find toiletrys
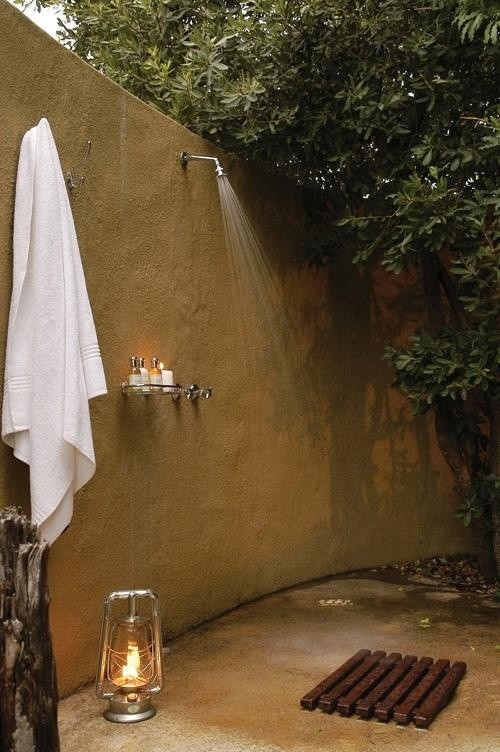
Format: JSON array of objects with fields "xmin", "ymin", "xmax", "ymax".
[
  {"xmin": 128, "ymin": 356, "xmax": 142, "ymax": 392},
  {"xmin": 139, "ymin": 359, "xmax": 149, "ymax": 391},
  {"xmin": 150, "ymin": 358, "xmax": 162, "ymax": 392}
]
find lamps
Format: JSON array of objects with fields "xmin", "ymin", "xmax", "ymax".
[{"xmin": 96, "ymin": 587, "xmax": 164, "ymax": 723}]
